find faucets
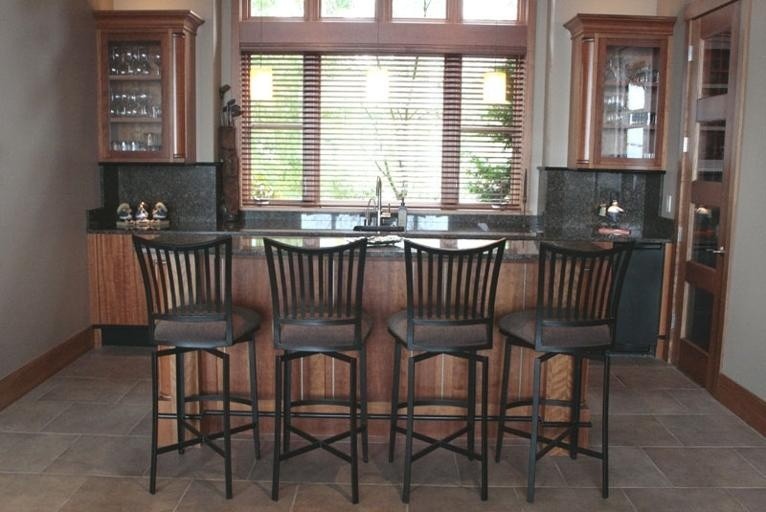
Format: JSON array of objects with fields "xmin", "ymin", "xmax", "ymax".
[{"xmin": 375, "ymin": 175, "xmax": 382, "ymax": 226}]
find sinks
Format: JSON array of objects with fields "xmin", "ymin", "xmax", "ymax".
[{"xmin": 353, "ymin": 225, "xmax": 404, "ymax": 232}]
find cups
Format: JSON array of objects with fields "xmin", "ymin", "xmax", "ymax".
[
  {"xmin": 132, "ymin": 47, "xmax": 140, "ymax": 75},
  {"xmin": 129, "ymin": 89, "xmax": 136, "ymax": 118},
  {"xmin": 120, "ymin": 136, "xmax": 128, "ymax": 151},
  {"xmin": 152, "ymin": 103, "xmax": 161, "ymax": 118},
  {"xmin": 138, "ymin": 47, "xmax": 149, "ymax": 75},
  {"xmin": 112, "ymin": 136, "xmax": 120, "ymax": 151},
  {"xmin": 122, "ymin": 89, "xmax": 129, "ymax": 118},
  {"xmin": 110, "ymin": 88, "xmax": 121, "ymax": 117},
  {"xmin": 111, "ymin": 46, "xmax": 121, "ymax": 75},
  {"xmin": 130, "ymin": 137, "xmax": 138, "ymax": 152},
  {"xmin": 152, "ymin": 48, "xmax": 161, "ymax": 76},
  {"xmin": 136, "ymin": 88, "xmax": 152, "ymax": 118},
  {"xmin": 122, "ymin": 46, "xmax": 132, "ymax": 75}
]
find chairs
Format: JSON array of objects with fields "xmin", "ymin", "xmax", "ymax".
[
  {"xmin": 261, "ymin": 235, "xmax": 375, "ymax": 505},
  {"xmin": 493, "ymin": 238, "xmax": 638, "ymax": 504},
  {"xmin": 386, "ymin": 235, "xmax": 508, "ymax": 503},
  {"xmin": 132, "ymin": 231, "xmax": 264, "ymax": 499}
]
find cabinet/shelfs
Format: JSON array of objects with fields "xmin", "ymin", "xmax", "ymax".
[
  {"xmin": 94, "ymin": 8, "xmax": 205, "ymax": 165},
  {"xmin": 88, "ymin": 229, "xmax": 158, "ymax": 349},
  {"xmin": 565, "ymin": 13, "xmax": 666, "ymax": 169}
]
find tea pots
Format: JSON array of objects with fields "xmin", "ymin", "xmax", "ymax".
[{"xmin": 143, "ymin": 132, "xmax": 159, "ymax": 152}]
